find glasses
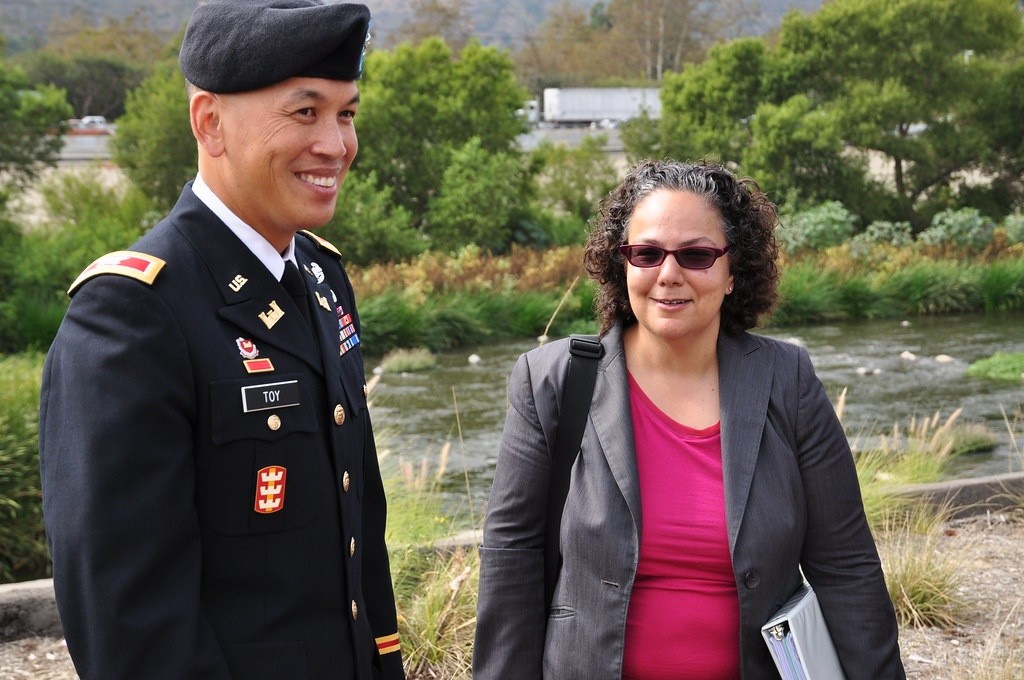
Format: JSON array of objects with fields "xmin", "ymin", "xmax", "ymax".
[{"xmin": 617, "ymin": 243, "xmax": 734, "ymax": 268}]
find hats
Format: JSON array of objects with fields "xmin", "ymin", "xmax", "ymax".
[{"xmin": 179, "ymin": 0, "xmax": 370, "ymax": 93}]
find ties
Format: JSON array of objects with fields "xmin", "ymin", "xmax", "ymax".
[{"xmin": 283, "ymin": 261, "xmax": 320, "ymax": 349}]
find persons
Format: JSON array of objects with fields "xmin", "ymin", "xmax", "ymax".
[
  {"xmin": 468, "ymin": 157, "xmax": 910, "ymax": 680},
  {"xmin": 36, "ymin": 0, "xmax": 408, "ymax": 680}
]
